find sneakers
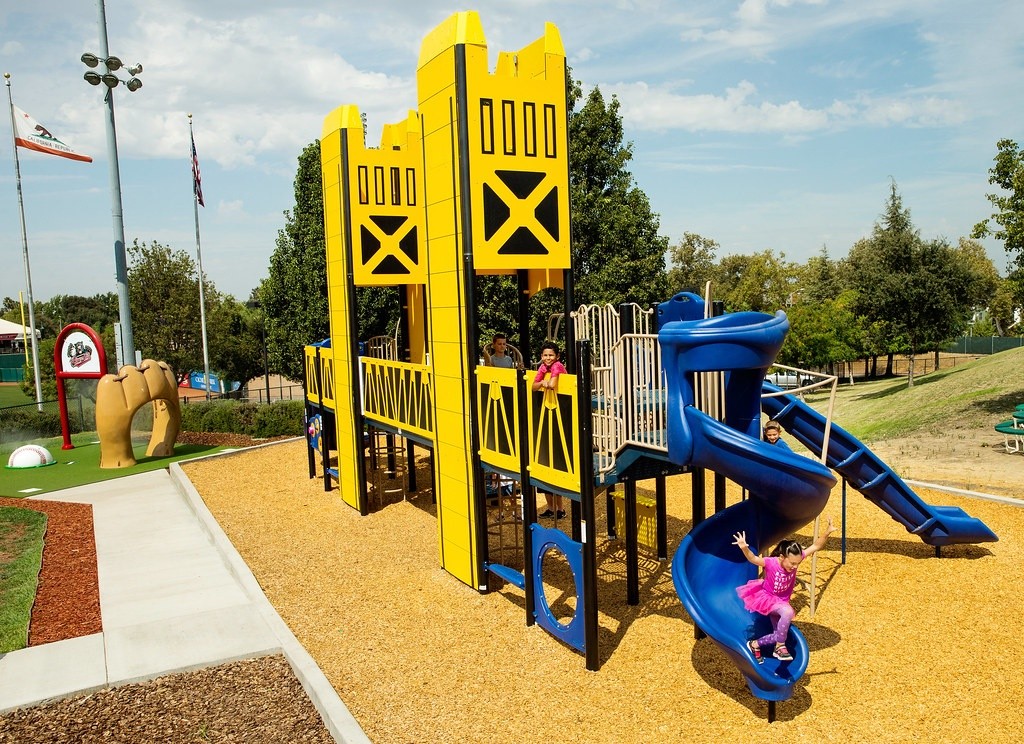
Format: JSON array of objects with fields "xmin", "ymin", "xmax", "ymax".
[
  {"xmin": 538, "ymin": 509, "xmax": 555, "ymax": 519},
  {"xmin": 550, "ymin": 509, "xmax": 566, "ymax": 521},
  {"xmin": 773, "ymin": 644, "xmax": 793, "ymax": 660},
  {"xmin": 746, "ymin": 640, "xmax": 764, "ymax": 664}
]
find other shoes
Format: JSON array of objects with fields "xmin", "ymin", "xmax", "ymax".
[
  {"xmin": 513, "ymin": 515, "xmax": 521, "ymax": 520},
  {"xmin": 496, "ymin": 515, "xmax": 505, "ymax": 521}
]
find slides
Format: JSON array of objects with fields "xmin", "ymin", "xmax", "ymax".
[
  {"xmin": 750, "ymin": 376, "xmax": 1003, "ymax": 557},
  {"xmin": 671, "ymin": 403, "xmax": 839, "ymax": 702}
]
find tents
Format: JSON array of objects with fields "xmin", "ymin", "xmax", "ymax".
[{"xmin": 1, "ymin": 316, "xmax": 41, "ymax": 342}]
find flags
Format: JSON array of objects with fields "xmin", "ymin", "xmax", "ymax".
[
  {"xmin": 189, "ymin": 122, "xmax": 205, "ymax": 209},
  {"xmin": 13, "ymin": 100, "xmax": 95, "ymax": 163}
]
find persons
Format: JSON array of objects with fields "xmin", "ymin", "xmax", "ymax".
[
  {"xmin": 732, "ymin": 518, "xmax": 838, "ymax": 668},
  {"xmin": 533, "ymin": 341, "xmax": 575, "ymax": 396},
  {"xmin": 763, "ymin": 421, "xmax": 794, "ymax": 456},
  {"xmin": 483, "ymin": 331, "xmax": 528, "ymax": 382},
  {"xmin": 485, "ymin": 469, "xmax": 525, "ymax": 524},
  {"xmin": 537, "ymin": 486, "xmax": 569, "ymax": 522}
]
